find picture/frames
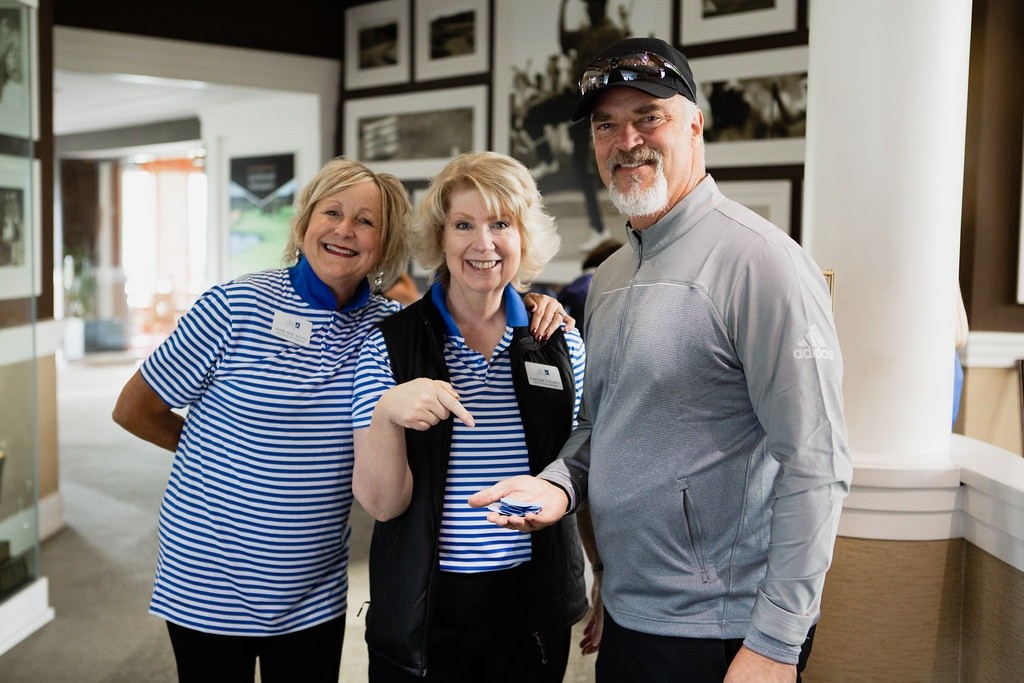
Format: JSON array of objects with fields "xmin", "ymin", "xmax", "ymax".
[
  {"xmin": 671, "ymin": 0, "xmax": 808, "ymax": 60},
  {"xmin": 412, "ymin": 0, "xmax": 495, "ymax": 80},
  {"xmin": 338, "ymin": 0, "xmax": 411, "ymax": 94},
  {"xmin": 705, "ymin": 162, "xmax": 804, "ymax": 245},
  {"xmin": 410, "ymin": 185, "xmax": 433, "ymax": 293},
  {"xmin": 0, "ymin": 0, "xmax": 54, "ymax": 331},
  {"xmin": 334, "ymin": 76, "xmax": 493, "ymax": 185}
]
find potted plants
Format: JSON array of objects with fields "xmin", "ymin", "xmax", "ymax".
[{"xmin": 64, "ymin": 245, "xmax": 103, "ymax": 348}]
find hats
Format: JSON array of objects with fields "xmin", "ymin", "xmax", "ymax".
[{"xmin": 572, "ymin": 38, "xmax": 696, "ymax": 123}]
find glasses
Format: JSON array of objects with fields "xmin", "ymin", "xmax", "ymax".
[{"xmin": 578, "ymin": 51, "xmax": 697, "ymax": 104}]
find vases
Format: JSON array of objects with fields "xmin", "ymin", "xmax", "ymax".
[{"xmin": 96, "ymin": 321, "xmax": 128, "ymax": 351}]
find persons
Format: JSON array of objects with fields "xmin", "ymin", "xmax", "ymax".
[
  {"xmin": 501, "ymin": 1, "xmax": 809, "ymax": 163},
  {"xmin": 353, "ymin": 152, "xmax": 606, "ymax": 683},
  {"xmin": 467, "ymin": 37, "xmax": 853, "ymax": 683},
  {"xmin": 111, "ymin": 156, "xmax": 578, "ymax": 683}
]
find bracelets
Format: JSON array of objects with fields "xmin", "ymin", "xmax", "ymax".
[{"xmin": 593, "ymin": 563, "xmax": 604, "ymax": 572}]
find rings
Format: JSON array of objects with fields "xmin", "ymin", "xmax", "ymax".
[{"xmin": 555, "ymin": 311, "xmax": 565, "ymax": 318}]
